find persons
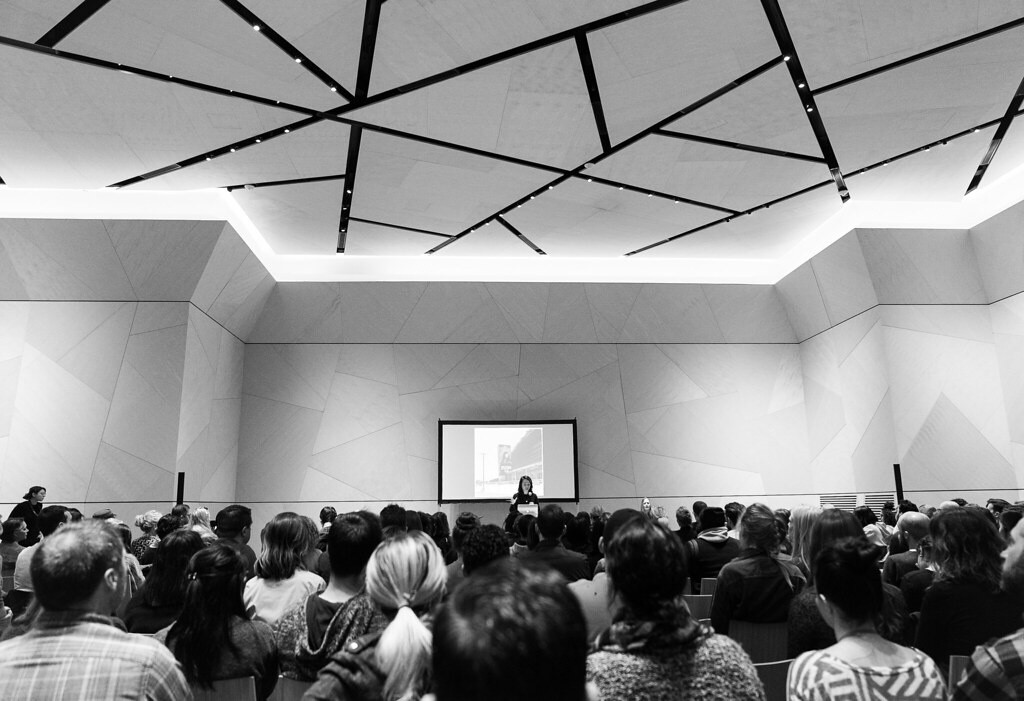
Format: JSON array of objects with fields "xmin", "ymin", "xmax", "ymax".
[
  {"xmin": 68, "ymin": 509, "xmax": 83, "ymax": 522},
  {"xmin": 509, "ymin": 475, "xmax": 540, "ymax": 512},
  {"xmin": 209, "ymin": 505, "xmax": 257, "ymax": 582},
  {"xmin": 7, "ymin": 485, "xmax": 46, "ymax": 546},
  {"xmin": 139, "ymin": 513, "xmax": 185, "ymax": 577},
  {"xmin": 92, "ymin": 508, "xmax": 116, "ymax": 527},
  {"xmin": 0, "ymin": 519, "xmax": 195, "ymax": 700},
  {"xmin": 292, "ymin": 503, "xmax": 611, "ymax": 583},
  {"xmin": 668, "ymin": 498, "xmax": 1024, "ymax": 609},
  {"xmin": 13, "ymin": 506, "xmax": 75, "ymax": 593},
  {"xmin": 123, "ymin": 530, "xmax": 213, "ymax": 634},
  {"xmin": 919, "ymin": 509, "xmax": 1017, "ymax": 686},
  {"xmin": 113, "ymin": 524, "xmax": 146, "ymax": 620},
  {"xmin": 297, "ymin": 530, "xmax": 450, "ymax": 701},
  {"xmin": 784, "ymin": 536, "xmax": 948, "ymax": 701},
  {"xmin": 154, "ymin": 544, "xmax": 279, "ymax": 701},
  {"xmin": 785, "ymin": 507, "xmax": 917, "ymax": 659},
  {"xmin": 708, "ymin": 504, "xmax": 806, "ymax": 665},
  {"xmin": 949, "ymin": 518, "xmax": 1024, "ymax": 701},
  {"xmin": 586, "ymin": 515, "xmax": 768, "ymax": 701},
  {"xmin": 420, "ymin": 558, "xmax": 602, "ymax": 701},
  {"xmin": 655, "ymin": 507, "xmax": 668, "ymax": 524},
  {"xmin": 565, "ymin": 508, "xmax": 649, "ymax": 651},
  {"xmin": 640, "ymin": 497, "xmax": 658, "ymax": 520},
  {"xmin": 0, "ymin": 555, "xmax": 11, "ymax": 636},
  {"xmin": 185, "ymin": 507, "xmax": 218, "ymax": 547},
  {"xmin": 172, "ymin": 504, "xmax": 191, "ymax": 529},
  {"xmin": 131, "ymin": 510, "xmax": 164, "ymax": 562},
  {"xmin": 269, "ymin": 511, "xmax": 396, "ymax": 681},
  {"xmin": 241, "ymin": 511, "xmax": 329, "ymax": 678}
]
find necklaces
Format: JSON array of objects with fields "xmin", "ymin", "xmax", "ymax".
[{"xmin": 838, "ymin": 629, "xmax": 878, "ymax": 640}]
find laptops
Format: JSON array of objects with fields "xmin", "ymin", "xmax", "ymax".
[{"xmin": 518, "ymin": 504, "xmax": 538, "ymax": 517}]
